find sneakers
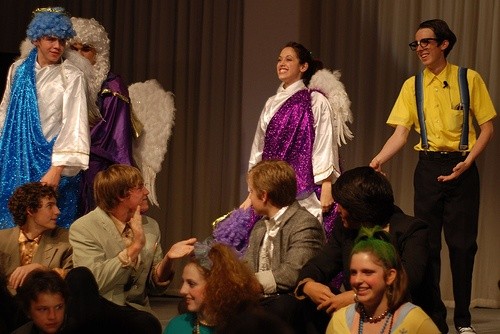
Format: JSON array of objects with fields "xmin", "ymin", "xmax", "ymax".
[{"xmin": 457, "ymin": 325, "xmax": 477, "ymax": 334}]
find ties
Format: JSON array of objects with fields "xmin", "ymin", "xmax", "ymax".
[
  {"xmin": 124, "ymin": 223, "xmax": 138, "ymax": 267},
  {"xmin": 22, "ymin": 241, "xmax": 36, "ymax": 266}
]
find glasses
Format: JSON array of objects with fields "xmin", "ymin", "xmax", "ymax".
[{"xmin": 409, "ymin": 38, "xmax": 437, "ymax": 51}]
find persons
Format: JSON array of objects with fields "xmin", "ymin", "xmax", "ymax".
[
  {"xmin": 368, "ymin": 19, "xmax": 496, "ymax": 334},
  {"xmin": 211, "ymin": 42, "xmax": 354, "ymax": 260},
  {"xmin": 10, "ymin": 265, "xmax": 70, "ymax": 334},
  {"xmin": 66, "ymin": 17, "xmax": 139, "ymax": 221},
  {"xmin": 68, "ymin": 164, "xmax": 197, "ymax": 309},
  {"xmin": 0, "ymin": 7, "xmax": 91, "ymax": 230},
  {"xmin": 325, "ymin": 225, "xmax": 443, "ymax": 334},
  {"xmin": 259, "ymin": 166, "xmax": 449, "ymax": 334},
  {"xmin": 240, "ymin": 160, "xmax": 325, "ymax": 299},
  {"xmin": 0, "ymin": 181, "xmax": 73, "ymax": 334},
  {"xmin": 161, "ymin": 239, "xmax": 264, "ymax": 334}
]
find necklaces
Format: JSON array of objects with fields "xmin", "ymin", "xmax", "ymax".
[
  {"xmin": 357, "ymin": 302, "xmax": 397, "ymax": 334},
  {"xmin": 192, "ymin": 315, "xmax": 219, "ymax": 334}
]
